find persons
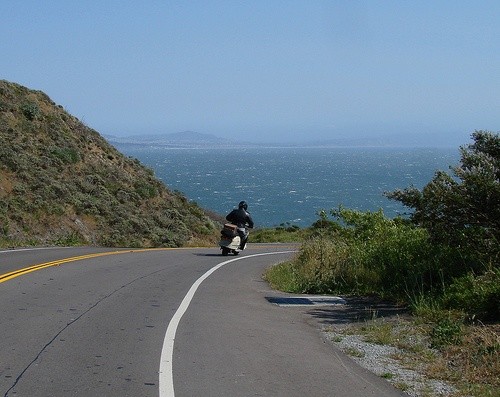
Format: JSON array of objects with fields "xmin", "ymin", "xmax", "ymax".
[{"xmin": 226, "ymin": 201, "xmax": 254, "ymax": 250}]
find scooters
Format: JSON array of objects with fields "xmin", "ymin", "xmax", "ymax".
[{"xmin": 219, "ymin": 221, "xmax": 250, "ymax": 256}]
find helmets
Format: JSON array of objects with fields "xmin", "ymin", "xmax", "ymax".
[{"xmin": 239, "ymin": 200, "xmax": 247, "ymax": 210}]
